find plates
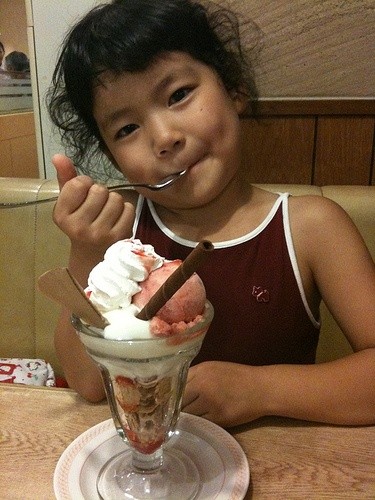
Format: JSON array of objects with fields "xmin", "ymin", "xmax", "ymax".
[{"xmin": 53, "ymin": 412, "xmax": 250, "ymax": 500}]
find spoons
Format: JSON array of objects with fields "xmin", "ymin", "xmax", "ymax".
[{"xmin": 107, "ymin": 168, "xmax": 188, "ymax": 193}]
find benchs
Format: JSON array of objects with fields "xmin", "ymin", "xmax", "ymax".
[{"xmin": 0, "ymin": 175, "xmax": 375, "ymax": 387}]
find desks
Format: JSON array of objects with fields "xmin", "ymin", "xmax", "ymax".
[{"xmin": 0, "ymin": 379, "xmax": 375, "ymax": 498}]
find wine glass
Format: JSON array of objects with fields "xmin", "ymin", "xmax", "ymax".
[{"xmin": 69, "ymin": 298, "xmax": 215, "ymax": 500}]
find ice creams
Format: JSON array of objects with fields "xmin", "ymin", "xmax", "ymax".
[{"xmin": 37, "ymin": 237, "xmax": 213, "ymax": 455}]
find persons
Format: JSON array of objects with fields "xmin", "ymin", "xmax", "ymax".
[
  {"xmin": 51, "ymin": 0, "xmax": 375, "ymax": 429},
  {"xmin": 0, "ymin": 41, "xmax": 32, "ymax": 86}
]
